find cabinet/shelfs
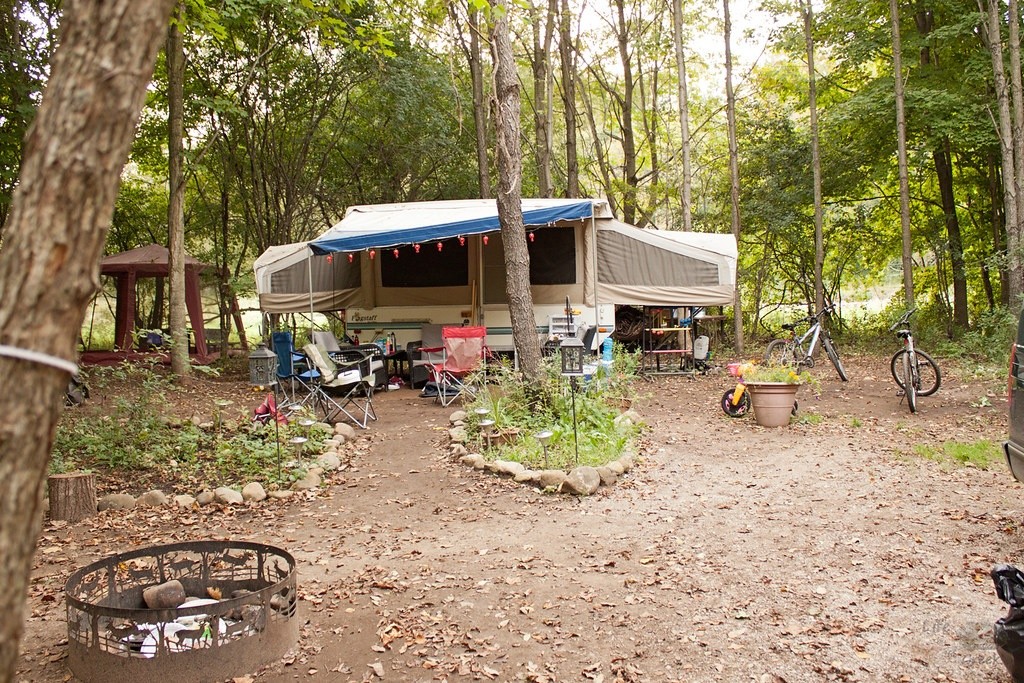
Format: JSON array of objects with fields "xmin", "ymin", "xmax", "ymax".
[{"xmin": 640, "ymin": 304, "xmax": 697, "ymax": 378}]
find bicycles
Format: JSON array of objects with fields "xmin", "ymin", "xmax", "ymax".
[
  {"xmin": 887, "ymin": 306, "xmax": 941, "ymax": 415},
  {"xmin": 764, "ymin": 302, "xmax": 850, "ymax": 382}
]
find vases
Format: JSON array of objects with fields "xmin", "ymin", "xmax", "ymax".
[{"xmin": 740, "ymin": 381, "xmax": 801, "ymax": 428}]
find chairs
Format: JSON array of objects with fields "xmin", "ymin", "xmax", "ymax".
[
  {"xmin": 300, "ymin": 341, "xmax": 379, "ymax": 431},
  {"xmin": 269, "ymin": 332, "xmax": 319, "ymax": 402},
  {"xmin": 312, "ymin": 330, "xmax": 393, "ymax": 397}
]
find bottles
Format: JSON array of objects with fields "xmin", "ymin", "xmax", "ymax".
[
  {"xmin": 383, "ymin": 331, "xmax": 396, "ymax": 356},
  {"xmin": 604, "ymin": 336, "xmax": 612, "ymax": 361}
]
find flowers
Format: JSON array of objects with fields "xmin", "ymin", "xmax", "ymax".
[{"xmin": 737, "ymin": 362, "xmax": 817, "ymax": 387}]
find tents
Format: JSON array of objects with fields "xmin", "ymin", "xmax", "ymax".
[
  {"xmin": 306, "ymin": 197, "xmax": 609, "ymax": 410},
  {"xmin": 75, "ymin": 243, "xmax": 248, "ymax": 369}
]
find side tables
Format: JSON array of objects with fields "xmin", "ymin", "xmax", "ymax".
[{"xmin": 416, "ymin": 325, "xmax": 490, "ymax": 408}]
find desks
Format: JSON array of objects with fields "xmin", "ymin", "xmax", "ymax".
[{"xmin": 381, "ymin": 350, "xmax": 406, "ymax": 393}]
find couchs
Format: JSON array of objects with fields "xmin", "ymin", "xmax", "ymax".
[{"xmin": 406, "ymin": 321, "xmax": 464, "ymax": 390}]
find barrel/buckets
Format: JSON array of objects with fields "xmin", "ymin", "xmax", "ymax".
[{"xmin": 694, "ymin": 336, "xmax": 710, "ymax": 359}]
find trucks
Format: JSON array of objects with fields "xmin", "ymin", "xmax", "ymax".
[{"xmin": 249, "ymin": 197, "xmax": 740, "ymax": 363}]
